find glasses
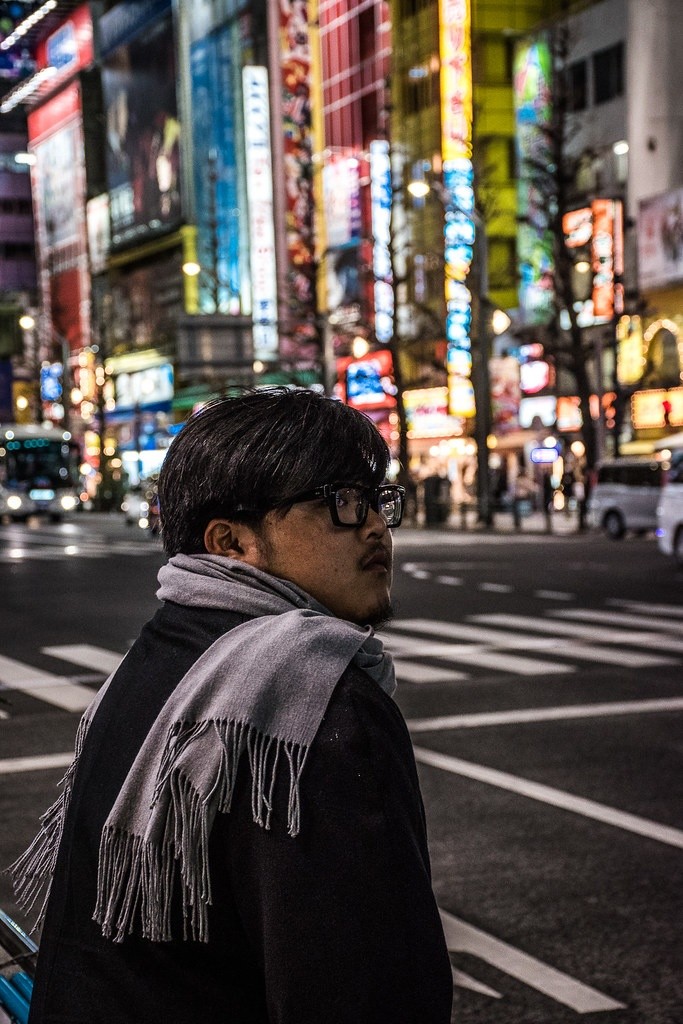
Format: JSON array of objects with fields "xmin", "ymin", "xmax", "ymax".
[{"xmin": 263, "ymin": 482, "xmax": 406, "ymax": 529}]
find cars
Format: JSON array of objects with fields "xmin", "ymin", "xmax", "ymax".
[{"xmin": 82, "ymin": 467, "xmax": 162, "ymax": 537}]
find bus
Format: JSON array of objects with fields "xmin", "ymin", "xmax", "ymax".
[{"xmin": 0, "ymin": 422, "xmax": 83, "ymax": 525}]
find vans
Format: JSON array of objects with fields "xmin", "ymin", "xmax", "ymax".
[{"xmin": 586, "ymin": 450, "xmax": 683, "ymax": 568}]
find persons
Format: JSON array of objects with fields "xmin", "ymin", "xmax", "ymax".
[
  {"xmin": 8, "ymin": 382, "xmax": 457, "ymax": 1023},
  {"xmin": 488, "ymin": 462, "xmax": 576, "ymax": 536}
]
